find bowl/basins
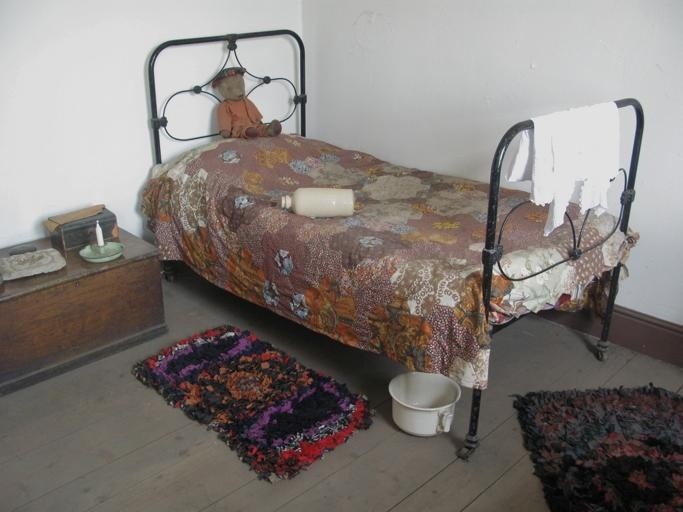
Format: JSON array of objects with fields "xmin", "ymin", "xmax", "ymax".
[{"xmin": 387, "ymin": 371, "xmax": 462, "ymax": 438}]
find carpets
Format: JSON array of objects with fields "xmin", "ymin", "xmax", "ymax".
[
  {"xmin": 507, "ymin": 382, "xmax": 683, "ymax": 512},
  {"xmin": 131, "ymin": 324, "xmax": 378, "ymax": 485}
]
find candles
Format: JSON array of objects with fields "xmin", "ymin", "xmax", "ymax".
[{"xmin": 95, "ymin": 220, "xmax": 104, "ymax": 247}]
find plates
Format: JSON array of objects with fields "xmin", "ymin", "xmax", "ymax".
[{"xmin": 78, "ymin": 241, "xmax": 126, "ymax": 263}]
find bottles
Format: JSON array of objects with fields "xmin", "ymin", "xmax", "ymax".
[{"xmin": 281, "ymin": 187, "xmax": 355, "ymax": 219}]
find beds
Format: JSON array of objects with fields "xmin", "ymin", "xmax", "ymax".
[{"xmin": 140, "ymin": 28, "xmax": 645, "ymax": 461}]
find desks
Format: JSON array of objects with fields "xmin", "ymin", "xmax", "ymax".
[{"xmin": 0, "ymin": 226, "xmax": 168, "ymax": 398}]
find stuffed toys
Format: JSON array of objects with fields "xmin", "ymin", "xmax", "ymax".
[{"xmin": 212, "ymin": 67, "xmax": 282, "ymax": 138}]
